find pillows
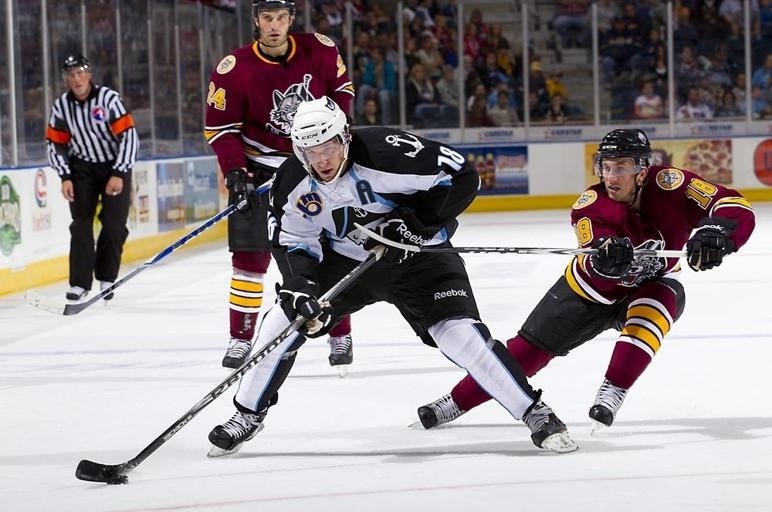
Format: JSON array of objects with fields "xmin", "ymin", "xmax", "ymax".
[
  {"xmin": 251, "ymin": 0, "xmax": 296, "ymax": 19},
  {"xmin": 594, "ymin": 129, "xmax": 653, "ymax": 177},
  {"xmin": 291, "ymin": 95, "xmax": 353, "ymax": 165},
  {"xmin": 62, "ymin": 54, "xmax": 92, "ymax": 80}
]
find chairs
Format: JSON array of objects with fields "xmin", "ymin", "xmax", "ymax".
[
  {"xmin": 328, "ymin": 335, "xmax": 352, "ymax": 365},
  {"xmin": 209, "ymin": 407, "xmax": 267, "ymax": 450},
  {"xmin": 590, "ymin": 378, "xmax": 628, "ymax": 426},
  {"xmin": 67, "ymin": 286, "xmax": 88, "ymax": 299},
  {"xmin": 522, "ymin": 398, "xmax": 567, "ymax": 448},
  {"xmin": 101, "ymin": 281, "xmax": 113, "ymax": 299},
  {"xmin": 222, "ymin": 337, "xmax": 251, "ymax": 368},
  {"xmin": 418, "ymin": 392, "xmax": 466, "ymax": 428}
]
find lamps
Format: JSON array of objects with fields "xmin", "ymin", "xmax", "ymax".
[
  {"xmin": 352, "ymin": 40, "xmax": 462, "ymax": 128},
  {"xmin": 557, "ymin": 1, "xmax": 698, "ymax": 125}
]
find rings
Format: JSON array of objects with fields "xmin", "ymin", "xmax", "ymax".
[{"xmin": 112, "ymin": 192, "xmax": 118, "ymax": 195}]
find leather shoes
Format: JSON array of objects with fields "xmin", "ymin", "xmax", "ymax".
[
  {"xmin": 276, "ymin": 274, "xmax": 335, "ymax": 338},
  {"xmin": 590, "ymin": 236, "xmax": 633, "ymax": 279},
  {"xmin": 364, "ymin": 205, "xmax": 441, "ymax": 266},
  {"xmin": 687, "ymin": 217, "xmax": 736, "ymax": 271},
  {"xmin": 224, "ymin": 171, "xmax": 257, "ymax": 219}
]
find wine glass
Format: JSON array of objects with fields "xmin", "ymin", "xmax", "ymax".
[{"xmin": 112, "ymin": 192, "xmax": 118, "ymax": 195}]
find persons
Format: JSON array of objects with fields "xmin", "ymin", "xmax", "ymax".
[
  {"xmin": 2, "ymin": 3, "xmax": 222, "ymax": 141},
  {"xmin": 288, "ymin": 3, "xmax": 573, "ymax": 125},
  {"xmin": 209, "ymin": 95, "xmax": 566, "ymax": 451},
  {"xmin": 205, "ymin": 2, "xmax": 352, "ymax": 369},
  {"xmin": 418, "ymin": 129, "xmax": 754, "ymax": 429},
  {"xmin": 551, "ymin": 3, "xmax": 769, "ymax": 119},
  {"xmin": 47, "ymin": 54, "xmax": 140, "ymax": 300}
]
